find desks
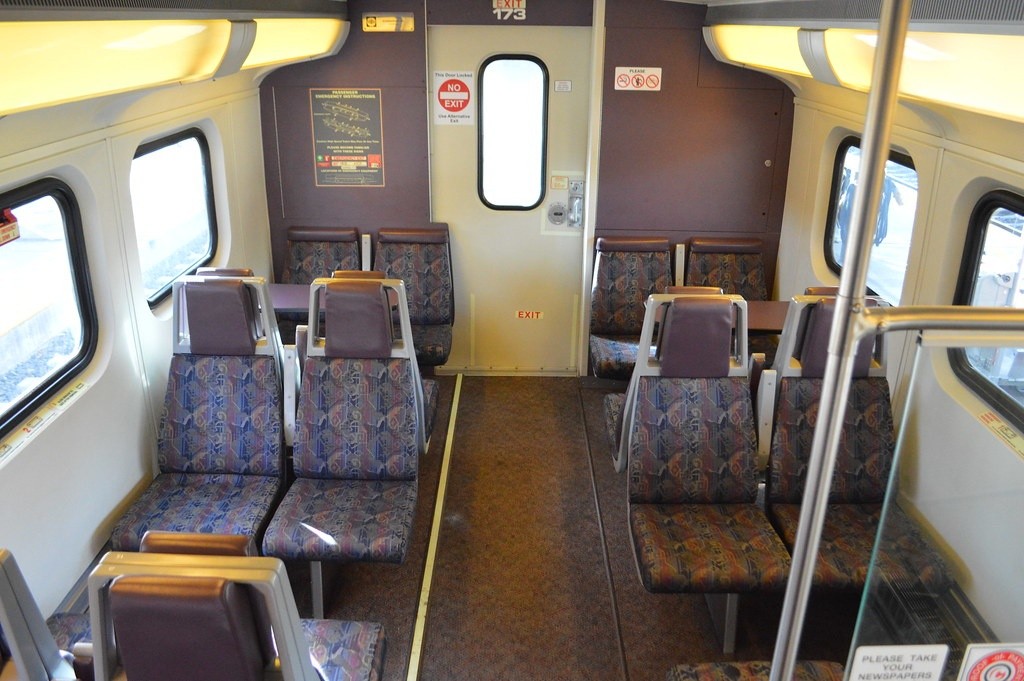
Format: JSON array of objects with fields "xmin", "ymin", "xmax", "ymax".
[{"xmin": 642, "ymin": 297, "xmax": 791, "ymax": 358}]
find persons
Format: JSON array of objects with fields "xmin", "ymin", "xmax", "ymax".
[{"xmin": 830, "ymin": 163, "xmax": 901, "ymax": 267}]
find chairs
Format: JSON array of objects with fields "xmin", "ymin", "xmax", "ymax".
[
  {"xmin": 602, "ymin": 284, "xmax": 745, "ymax": 474},
  {"xmin": 106, "ymin": 264, "xmax": 300, "ymax": 554},
  {"xmin": 275, "ymin": 227, "xmax": 363, "ymax": 344},
  {"xmin": 747, "ymin": 284, "xmax": 952, "ymax": 599},
  {"xmin": 260, "ymin": 270, "xmax": 444, "ymax": 569},
  {"xmin": 589, "ymin": 234, "xmax": 676, "ymax": 383},
  {"xmin": 370, "ymin": 226, "xmax": 457, "ymax": 366},
  {"xmin": 0, "ymin": 528, "xmax": 390, "ymax": 680},
  {"xmin": 683, "ymin": 236, "xmax": 781, "ymax": 370},
  {"xmin": 622, "ymin": 293, "xmax": 795, "ymax": 598}
]
